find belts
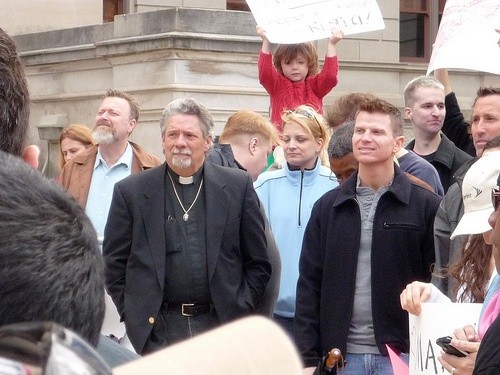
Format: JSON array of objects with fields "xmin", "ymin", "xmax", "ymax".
[{"xmin": 169, "ymin": 302, "xmax": 211, "ymax": 317}]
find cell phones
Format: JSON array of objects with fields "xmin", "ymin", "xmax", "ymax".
[{"xmin": 436, "ymin": 336, "xmax": 469, "ymax": 357}]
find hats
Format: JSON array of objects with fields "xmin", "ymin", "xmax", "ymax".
[{"xmin": 449, "ymin": 150, "xmax": 500, "ymax": 241}]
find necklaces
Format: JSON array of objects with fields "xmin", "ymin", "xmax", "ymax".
[{"xmin": 166, "ymin": 170, "xmax": 203, "ymax": 221}]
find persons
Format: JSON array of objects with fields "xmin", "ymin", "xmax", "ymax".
[
  {"xmin": 0, "ymin": 27, "xmax": 40, "ymax": 170},
  {"xmin": 255, "ymin": 26, "xmax": 343, "ymax": 134},
  {"xmin": 0, "ymin": 44, "xmax": 500, "ymax": 375}
]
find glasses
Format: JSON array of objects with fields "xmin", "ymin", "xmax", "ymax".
[
  {"xmin": 286, "ymin": 108, "xmax": 322, "ymax": 136},
  {"xmin": 490, "ymin": 188, "xmax": 500, "ymax": 209}
]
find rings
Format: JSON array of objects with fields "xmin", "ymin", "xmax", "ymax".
[{"xmin": 450, "ymin": 367, "xmax": 456, "ymax": 375}]
